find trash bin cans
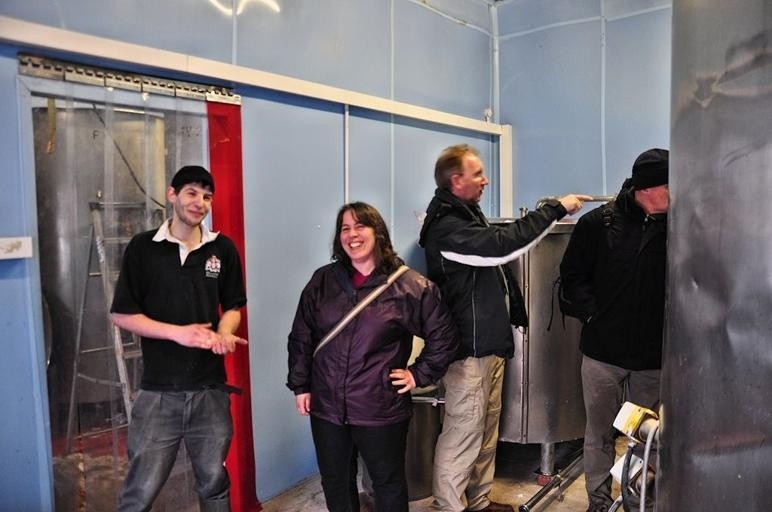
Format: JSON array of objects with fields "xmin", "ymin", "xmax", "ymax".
[{"xmin": 360, "ymin": 385, "xmax": 443, "ymax": 502}]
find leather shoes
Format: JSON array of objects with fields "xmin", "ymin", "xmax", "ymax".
[{"xmin": 468, "ymin": 502, "xmax": 514, "ymax": 512}]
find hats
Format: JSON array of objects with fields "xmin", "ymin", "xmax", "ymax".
[{"xmin": 632, "ymin": 149, "xmax": 668, "ymax": 191}]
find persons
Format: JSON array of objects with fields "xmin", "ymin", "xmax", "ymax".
[
  {"xmin": 285, "ymin": 201, "xmax": 456, "ymax": 511},
  {"xmin": 419, "ymin": 144, "xmax": 594, "ymax": 511},
  {"xmin": 109, "ymin": 166, "xmax": 247, "ymax": 511},
  {"xmin": 557, "ymin": 147, "xmax": 668, "ymax": 511}
]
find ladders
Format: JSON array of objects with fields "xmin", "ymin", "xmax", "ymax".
[{"xmin": 65, "ymin": 200, "xmax": 147, "ymax": 456}]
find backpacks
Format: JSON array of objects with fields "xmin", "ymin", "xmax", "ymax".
[{"xmin": 557, "ymin": 202, "xmax": 624, "ymax": 323}]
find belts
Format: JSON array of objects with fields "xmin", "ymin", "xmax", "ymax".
[{"xmin": 143, "ymin": 383, "xmax": 244, "ymax": 396}]
